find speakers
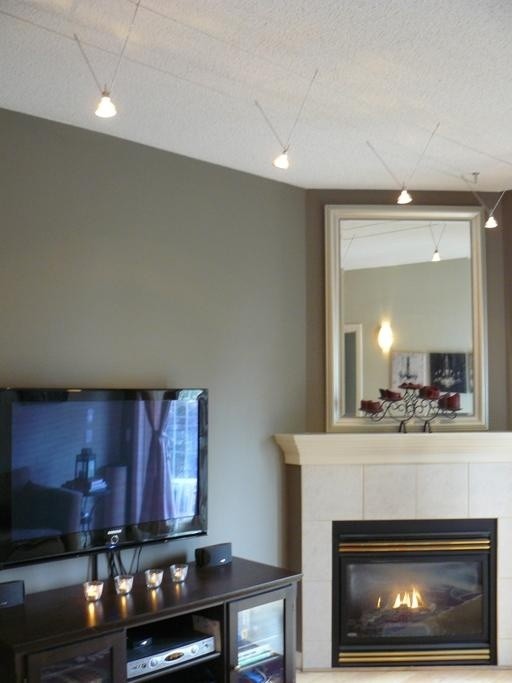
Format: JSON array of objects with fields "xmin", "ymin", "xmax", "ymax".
[
  {"xmin": 0, "ymin": 580, "xmax": 26, "ymax": 609},
  {"xmin": 195, "ymin": 543, "xmax": 232, "ymax": 570}
]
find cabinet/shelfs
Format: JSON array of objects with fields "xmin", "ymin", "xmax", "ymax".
[{"xmin": 0, "ymin": 557, "xmax": 303, "ymax": 683}]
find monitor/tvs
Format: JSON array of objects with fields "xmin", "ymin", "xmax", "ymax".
[{"xmin": 0, "ymin": 387, "xmax": 209, "ymax": 572}]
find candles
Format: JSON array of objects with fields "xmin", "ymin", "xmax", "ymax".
[
  {"xmin": 366, "ymin": 383, "xmax": 460, "ymax": 412},
  {"xmin": 361, "ymin": 400, "xmax": 371, "ymax": 409}
]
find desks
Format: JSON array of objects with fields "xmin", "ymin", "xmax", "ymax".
[{"xmin": 61, "ymin": 484, "xmax": 112, "ymax": 547}]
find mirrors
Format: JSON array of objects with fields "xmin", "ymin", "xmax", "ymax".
[{"xmin": 324, "ymin": 204, "xmax": 488, "ymax": 432}]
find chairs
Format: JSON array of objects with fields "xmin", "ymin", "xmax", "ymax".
[{"xmin": 0, "ymin": 466, "xmax": 83, "ymax": 559}]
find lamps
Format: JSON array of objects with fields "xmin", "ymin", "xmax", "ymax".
[
  {"xmin": 366, "ymin": 122, "xmax": 440, "ymax": 204},
  {"xmin": 74, "ymin": 0, "xmax": 140, "ymax": 117},
  {"xmin": 255, "ymin": 69, "xmax": 319, "ymax": 169},
  {"xmin": 461, "ymin": 175, "xmax": 512, "ymax": 229},
  {"xmin": 428, "ymin": 223, "xmax": 446, "ymax": 261}
]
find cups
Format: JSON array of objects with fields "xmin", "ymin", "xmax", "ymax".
[{"xmin": 83, "ymin": 563, "xmax": 188, "ymax": 602}]
